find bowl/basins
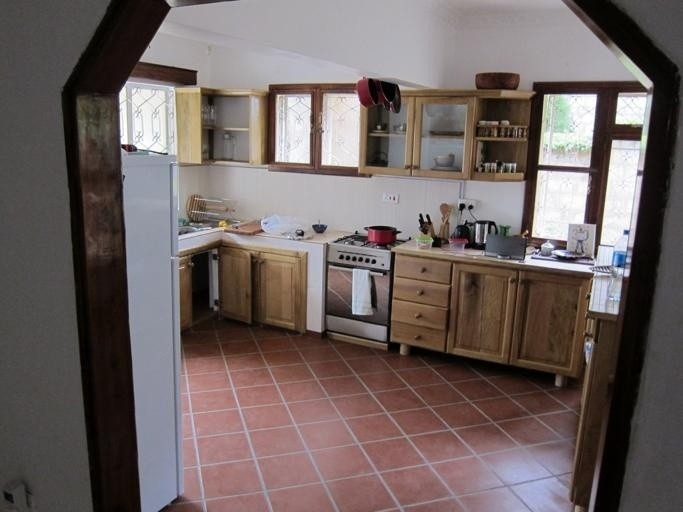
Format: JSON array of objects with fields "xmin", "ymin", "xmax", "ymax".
[
  {"xmin": 433, "ymin": 153, "xmax": 454, "ymax": 167},
  {"xmin": 312, "ymin": 224, "xmax": 326, "ymax": 233}
]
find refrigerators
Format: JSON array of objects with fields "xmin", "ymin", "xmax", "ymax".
[{"xmin": 121, "ymin": 154, "xmax": 185, "ymax": 512}]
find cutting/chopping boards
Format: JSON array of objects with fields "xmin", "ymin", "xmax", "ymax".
[{"xmin": 223, "ymin": 221, "xmax": 263, "ymax": 235}]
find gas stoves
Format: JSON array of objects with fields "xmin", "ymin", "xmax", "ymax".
[{"xmin": 328, "ymin": 233, "xmax": 407, "ymax": 270}]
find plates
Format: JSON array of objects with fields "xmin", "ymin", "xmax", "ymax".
[{"xmin": 551, "ymin": 250, "xmax": 585, "ymax": 260}]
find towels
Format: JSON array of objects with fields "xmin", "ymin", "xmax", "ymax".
[{"xmin": 351, "ymin": 268, "xmax": 376, "ymax": 317}]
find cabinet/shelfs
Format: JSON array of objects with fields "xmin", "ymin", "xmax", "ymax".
[
  {"xmin": 472, "ymin": 89, "xmax": 533, "ymax": 183},
  {"xmin": 178, "ymin": 240, "xmax": 216, "ymax": 335},
  {"xmin": 216, "ymin": 229, "xmax": 325, "ymax": 332},
  {"xmin": 175, "ymin": 87, "xmax": 269, "ymax": 167},
  {"xmin": 568, "ymin": 315, "xmax": 618, "ymax": 512},
  {"xmin": 388, "ymin": 238, "xmax": 453, "ymax": 356},
  {"xmin": 449, "ymin": 253, "xmax": 593, "ymax": 389},
  {"xmin": 359, "ymin": 89, "xmax": 472, "ymax": 182}
]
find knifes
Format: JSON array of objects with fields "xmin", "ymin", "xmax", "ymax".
[{"xmin": 418, "ymin": 213, "xmax": 430, "ymax": 227}]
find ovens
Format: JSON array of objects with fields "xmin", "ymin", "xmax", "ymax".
[{"xmin": 325, "ymin": 264, "xmax": 391, "ymax": 343}]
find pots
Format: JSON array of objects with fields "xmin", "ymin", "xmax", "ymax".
[
  {"xmin": 356, "ymin": 77, "xmax": 400, "ymax": 113},
  {"xmin": 363, "ymin": 226, "xmax": 401, "ymax": 244}
]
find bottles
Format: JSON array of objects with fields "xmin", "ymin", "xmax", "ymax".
[
  {"xmin": 476, "ymin": 160, "xmax": 517, "ymax": 173},
  {"xmin": 200, "ymin": 102, "xmax": 216, "ymax": 127},
  {"xmin": 605, "ymin": 229, "xmax": 629, "ymax": 278}
]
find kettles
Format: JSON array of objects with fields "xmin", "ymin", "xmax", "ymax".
[{"xmin": 473, "ymin": 220, "xmax": 497, "ymax": 250}]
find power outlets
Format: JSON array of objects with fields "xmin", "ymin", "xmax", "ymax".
[
  {"xmin": 383, "ymin": 192, "xmax": 399, "ymax": 203},
  {"xmin": 457, "ymin": 199, "xmax": 476, "ymax": 212}
]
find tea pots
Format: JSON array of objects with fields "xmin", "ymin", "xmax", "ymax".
[{"xmin": 220, "ymin": 133, "xmax": 238, "ymax": 159}]
